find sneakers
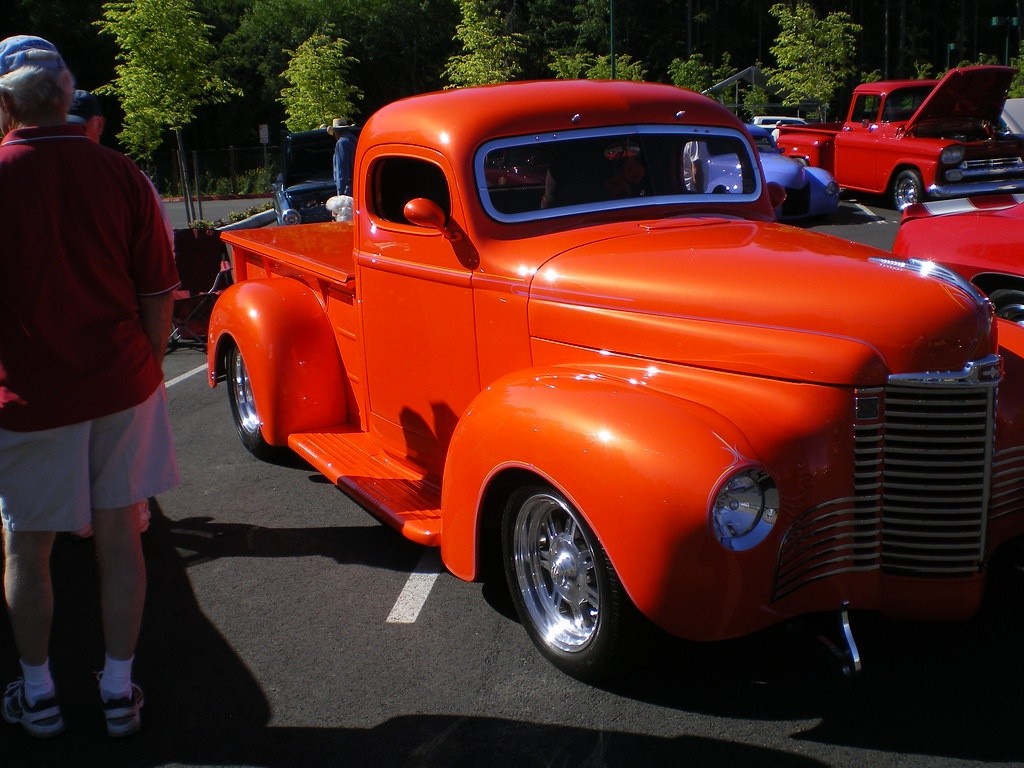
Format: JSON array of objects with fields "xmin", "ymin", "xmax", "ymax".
[
  {"xmin": 96, "ymin": 671, "xmax": 144, "ymax": 733},
  {"xmin": 0, "ymin": 679, "xmax": 63, "ymax": 734}
]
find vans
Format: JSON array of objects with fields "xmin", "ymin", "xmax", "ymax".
[{"xmin": 752, "ymin": 116, "xmax": 809, "ymax": 141}]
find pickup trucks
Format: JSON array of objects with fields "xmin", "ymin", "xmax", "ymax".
[
  {"xmin": 775, "ymin": 65, "xmax": 1024, "ymax": 216},
  {"xmin": 206, "ymin": 78, "xmax": 1024, "ymax": 685}
]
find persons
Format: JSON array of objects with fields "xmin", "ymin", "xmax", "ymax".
[
  {"xmin": 326, "ymin": 118, "xmax": 359, "ymax": 196},
  {"xmin": 63, "ymin": 83, "xmax": 152, "ymax": 540},
  {"xmin": 1, "ymin": 33, "xmax": 184, "ymax": 744}
]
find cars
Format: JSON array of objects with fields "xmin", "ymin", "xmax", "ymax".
[
  {"xmin": 894, "ymin": 192, "xmax": 1024, "ymax": 325},
  {"xmin": 678, "ymin": 122, "xmax": 841, "ymax": 222},
  {"xmin": 271, "ymin": 123, "xmax": 361, "ymax": 226}
]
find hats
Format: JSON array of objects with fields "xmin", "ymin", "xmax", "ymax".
[
  {"xmin": 327, "ymin": 118, "xmax": 355, "ymax": 136},
  {"xmin": 0, "ymin": 35, "xmax": 65, "ymax": 76},
  {"xmin": 64, "ymin": 90, "xmax": 103, "ymax": 125}
]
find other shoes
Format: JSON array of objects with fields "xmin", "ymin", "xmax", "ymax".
[
  {"xmin": 73, "ymin": 523, "xmax": 94, "ymax": 541},
  {"xmin": 137, "ymin": 499, "xmax": 151, "ymax": 532}
]
